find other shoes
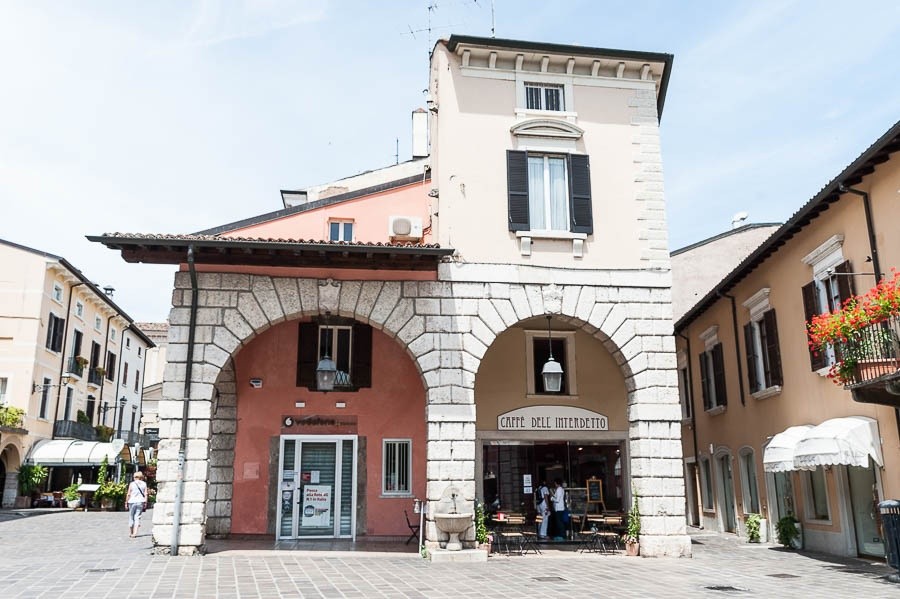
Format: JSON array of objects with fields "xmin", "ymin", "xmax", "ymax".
[
  {"xmin": 554, "ymin": 536, "xmax": 563, "ymax": 540},
  {"xmin": 540, "ymin": 535, "xmax": 550, "ymax": 539},
  {"xmin": 129, "ymin": 534, "xmax": 136, "ymax": 537}
]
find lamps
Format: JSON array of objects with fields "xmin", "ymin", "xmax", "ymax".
[
  {"xmin": 104, "ymin": 396, "xmax": 128, "ymax": 411},
  {"xmin": 317, "ymin": 311, "xmax": 336, "ymax": 391},
  {"xmin": 33, "ymin": 373, "xmax": 71, "ymax": 394},
  {"xmin": 542, "ymin": 316, "xmax": 563, "ymax": 391}
]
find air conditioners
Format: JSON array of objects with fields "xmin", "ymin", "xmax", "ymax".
[{"xmin": 387, "ymin": 216, "xmax": 423, "ymax": 241}]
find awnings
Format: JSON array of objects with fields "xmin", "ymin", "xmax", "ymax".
[{"xmin": 762, "ymin": 416, "xmax": 883, "ymax": 473}]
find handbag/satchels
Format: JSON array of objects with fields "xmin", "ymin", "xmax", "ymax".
[{"xmin": 562, "ymin": 510, "xmax": 569, "ymax": 523}]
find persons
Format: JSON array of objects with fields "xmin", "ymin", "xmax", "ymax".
[
  {"xmin": 534, "ymin": 478, "xmax": 567, "ymax": 539},
  {"xmin": 125, "ymin": 471, "xmax": 147, "ymax": 537}
]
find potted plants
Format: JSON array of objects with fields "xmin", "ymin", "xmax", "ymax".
[
  {"xmin": 621, "ymin": 484, "xmax": 640, "ymax": 556},
  {"xmin": 72, "ymin": 356, "xmax": 107, "ymax": 385},
  {"xmin": 15, "ymin": 453, "xmax": 156, "ymax": 509},
  {"xmin": 475, "ymin": 498, "xmax": 492, "ymax": 557},
  {"xmin": 0, "ymin": 403, "xmax": 24, "ymax": 428},
  {"xmin": 745, "ymin": 513, "xmax": 799, "ymax": 551},
  {"xmin": 77, "ymin": 410, "xmax": 116, "ymax": 443}
]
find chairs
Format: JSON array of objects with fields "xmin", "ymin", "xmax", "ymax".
[
  {"xmin": 497, "ymin": 511, "xmax": 622, "ymax": 556},
  {"xmin": 404, "ymin": 510, "xmax": 419, "ymax": 546}
]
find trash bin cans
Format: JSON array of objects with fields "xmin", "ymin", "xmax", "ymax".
[{"xmin": 877, "ymin": 499, "xmax": 900, "ymax": 570}]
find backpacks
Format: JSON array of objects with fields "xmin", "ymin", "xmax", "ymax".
[{"xmin": 536, "ymin": 487, "xmax": 544, "ymax": 505}]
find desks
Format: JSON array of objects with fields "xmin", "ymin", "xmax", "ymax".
[
  {"xmin": 587, "ymin": 519, "xmax": 622, "ymax": 552},
  {"xmin": 492, "ymin": 513, "xmax": 522, "ymax": 518},
  {"xmin": 568, "ymin": 514, "xmax": 604, "ymax": 541},
  {"xmin": 492, "ymin": 518, "xmax": 522, "ymax": 553}
]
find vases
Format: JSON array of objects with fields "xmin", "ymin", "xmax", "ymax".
[{"xmin": 854, "ymin": 358, "xmax": 897, "ymax": 383}]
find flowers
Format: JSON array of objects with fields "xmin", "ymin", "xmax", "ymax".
[{"xmin": 803, "ymin": 266, "xmax": 900, "ymax": 386}]
